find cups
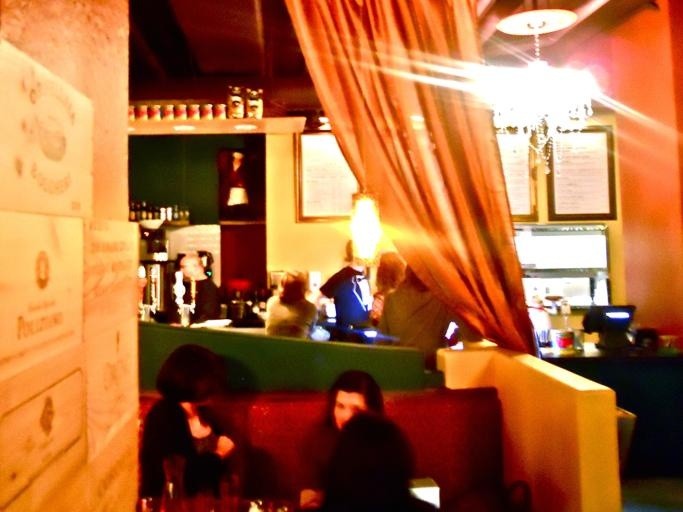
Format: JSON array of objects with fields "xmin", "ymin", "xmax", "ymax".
[
  {"xmin": 573, "ymin": 329, "xmax": 584, "ymax": 352},
  {"xmin": 535, "ymin": 329, "xmax": 551, "ymax": 348}
]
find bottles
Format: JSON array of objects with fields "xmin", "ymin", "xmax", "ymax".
[
  {"xmin": 229, "ymin": 291, "xmax": 245, "ymax": 320},
  {"xmin": 128, "ymin": 102, "xmax": 226, "ymax": 120},
  {"xmin": 129, "ymin": 199, "xmax": 192, "ymax": 222},
  {"xmin": 252, "ymin": 288, "xmax": 268, "ymax": 311},
  {"xmin": 226, "ymin": 83, "xmax": 264, "ymax": 120}
]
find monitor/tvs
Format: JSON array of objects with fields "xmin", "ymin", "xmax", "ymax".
[{"xmin": 582, "ymin": 305, "xmax": 636, "ymax": 333}]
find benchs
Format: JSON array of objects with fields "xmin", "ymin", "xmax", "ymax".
[{"xmin": 138, "ymin": 386, "xmax": 532, "ymax": 511}]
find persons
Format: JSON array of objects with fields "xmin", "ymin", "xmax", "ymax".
[
  {"xmin": 374, "ymin": 249, "xmax": 482, "ymax": 367},
  {"xmin": 300, "ymin": 369, "xmax": 383, "ymax": 506},
  {"xmin": 150, "ymin": 255, "xmax": 220, "ymax": 323},
  {"xmin": 370, "ymin": 253, "xmax": 406, "ymax": 324},
  {"xmin": 334, "ymin": 241, "xmax": 373, "ymax": 328},
  {"xmin": 265, "ymin": 269, "xmax": 318, "ymax": 338},
  {"xmin": 138, "ymin": 345, "xmax": 235, "ymax": 497},
  {"xmin": 306, "ymin": 411, "xmax": 435, "ymax": 508}
]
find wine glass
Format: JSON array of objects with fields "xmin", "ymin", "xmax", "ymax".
[{"xmin": 559, "ymin": 300, "xmax": 572, "ymax": 333}]
